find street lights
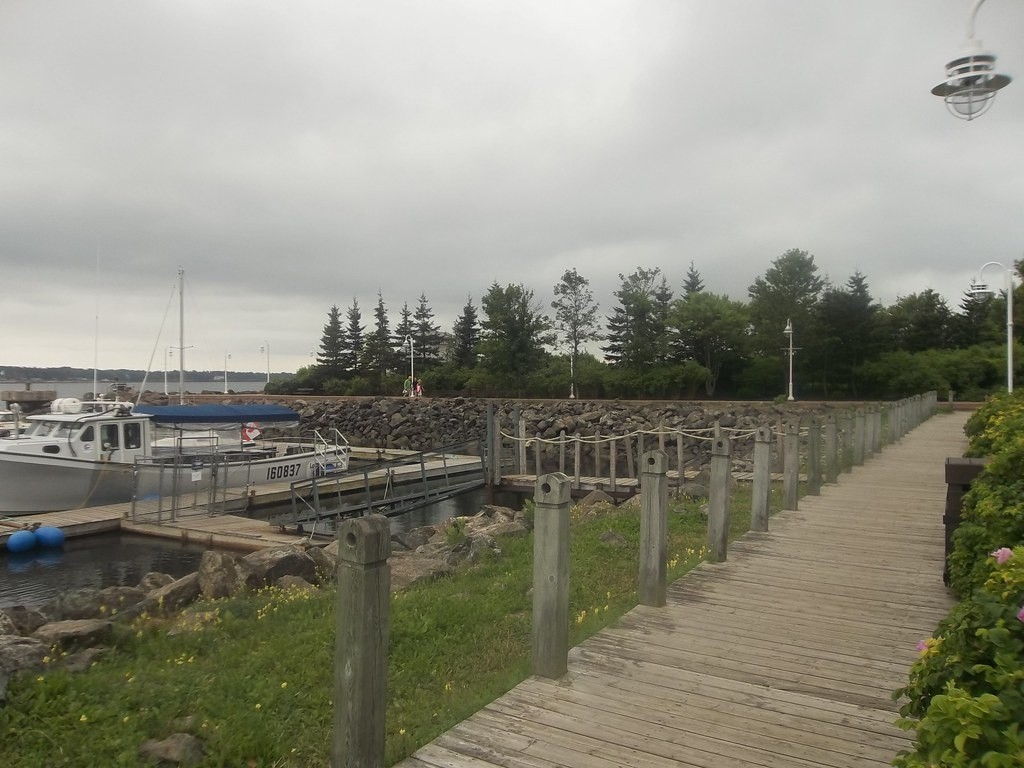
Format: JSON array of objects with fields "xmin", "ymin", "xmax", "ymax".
[
  {"xmin": 403, "ymin": 334, "xmax": 415, "ymax": 397},
  {"xmin": 224, "ymin": 351, "xmax": 231, "ymax": 394},
  {"xmin": 970, "ymin": 261, "xmax": 1013, "ymax": 397},
  {"xmin": 260, "ymin": 340, "xmax": 269, "ymax": 383},
  {"xmin": 781, "ymin": 317, "xmax": 796, "ymax": 401},
  {"xmin": 569, "ymin": 343, "xmax": 575, "ymax": 399}
]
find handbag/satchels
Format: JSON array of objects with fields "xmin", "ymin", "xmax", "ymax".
[{"xmin": 402, "ymin": 388, "xmax": 407, "ymax": 394}]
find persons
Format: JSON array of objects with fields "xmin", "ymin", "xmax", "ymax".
[{"xmin": 402, "ymin": 376, "xmax": 424, "ymax": 397}]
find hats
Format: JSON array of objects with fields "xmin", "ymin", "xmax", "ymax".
[
  {"xmin": 408, "ymin": 376, "xmax": 411, "ymax": 379},
  {"xmin": 417, "ymin": 379, "xmax": 422, "ymax": 382}
]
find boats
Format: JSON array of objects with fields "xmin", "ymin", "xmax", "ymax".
[{"xmin": 0, "ymin": 315, "xmax": 353, "ymax": 520}]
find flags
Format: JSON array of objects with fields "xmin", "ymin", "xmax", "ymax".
[{"xmin": 241, "ymin": 422, "xmax": 263, "ymax": 442}]
